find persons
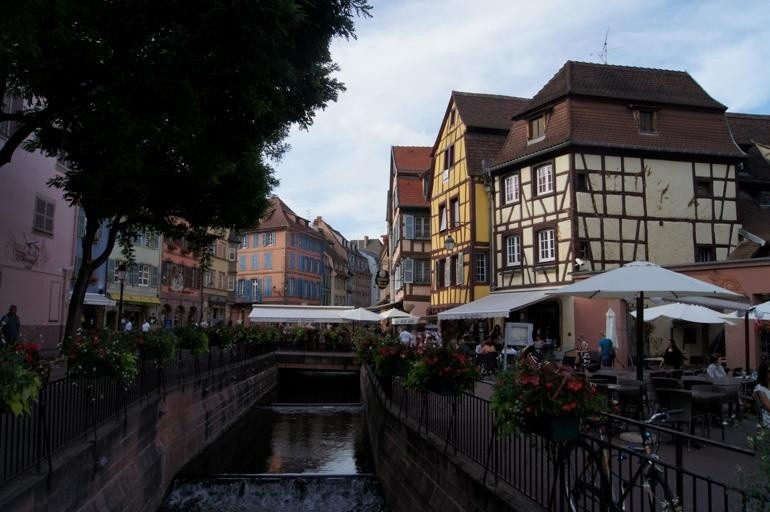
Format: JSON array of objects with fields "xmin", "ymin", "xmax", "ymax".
[
  {"xmin": 0, "ymin": 303, "xmax": 21, "ymax": 349},
  {"xmin": 117, "ymin": 316, "xmax": 352, "ymax": 338},
  {"xmin": 751, "ymin": 362, "xmax": 770, "ymax": 432},
  {"xmin": 364, "ymin": 323, "xmax": 617, "ymax": 372},
  {"xmin": 705, "ymin": 350, "xmax": 743, "ymax": 410},
  {"xmin": 656, "ymin": 338, "xmax": 686, "ymax": 370}
]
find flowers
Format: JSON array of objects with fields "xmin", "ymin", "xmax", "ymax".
[
  {"xmin": 0, "ymin": 321, "xmax": 272, "ymax": 420},
  {"xmin": 272, "ymin": 325, "xmax": 481, "ymax": 400},
  {"xmin": 484, "ymin": 351, "xmax": 625, "ymax": 441}
]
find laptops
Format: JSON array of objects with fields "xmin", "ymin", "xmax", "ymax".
[{"xmin": 732, "ymin": 368, "xmax": 742, "ymax": 377}]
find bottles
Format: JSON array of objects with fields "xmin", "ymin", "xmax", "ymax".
[{"xmin": 742, "ymin": 368, "xmax": 747, "ymax": 380}]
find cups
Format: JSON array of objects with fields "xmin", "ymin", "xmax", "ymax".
[{"xmin": 752, "ymin": 370, "xmax": 757, "ymax": 379}]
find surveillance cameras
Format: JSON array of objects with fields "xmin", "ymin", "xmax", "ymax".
[{"xmin": 575, "ymin": 257, "xmax": 584, "ymax": 267}]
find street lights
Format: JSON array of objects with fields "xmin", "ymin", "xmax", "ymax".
[{"xmin": 114, "ymin": 262, "xmax": 128, "ymax": 333}]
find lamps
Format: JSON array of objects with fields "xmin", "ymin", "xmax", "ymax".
[{"xmin": 443, "ymin": 221, "xmax": 473, "ymax": 251}]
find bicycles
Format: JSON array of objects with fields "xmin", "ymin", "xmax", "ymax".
[{"xmin": 563, "ymin": 405, "xmax": 684, "ymax": 512}]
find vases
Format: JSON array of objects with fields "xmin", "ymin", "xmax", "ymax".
[{"xmin": 524, "ymin": 413, "xmax": 582, "ymax": 453}]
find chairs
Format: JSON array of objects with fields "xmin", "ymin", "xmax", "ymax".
[{"xmin": 618, "ymin": 355, "xmax": 759, "ymax": 453}]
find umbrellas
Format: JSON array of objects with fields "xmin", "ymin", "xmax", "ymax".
[
  {"xmin": 377, "ymin": 307, "xmax": 413, "ymax": 320},
  {"xmin": 337, "ymin": 306, "xmax": 383, "ymax": 330},
  {"xmin": 716, "ymin": 297, "xmax": 770, "ymax": 330},
  {"xmin": 544, "ymin": 259, "xmax": 745, "ymax": 436},
  {"xmin": 628, "ymin": 302, "xmax": 737, "ymax": 339}
]
